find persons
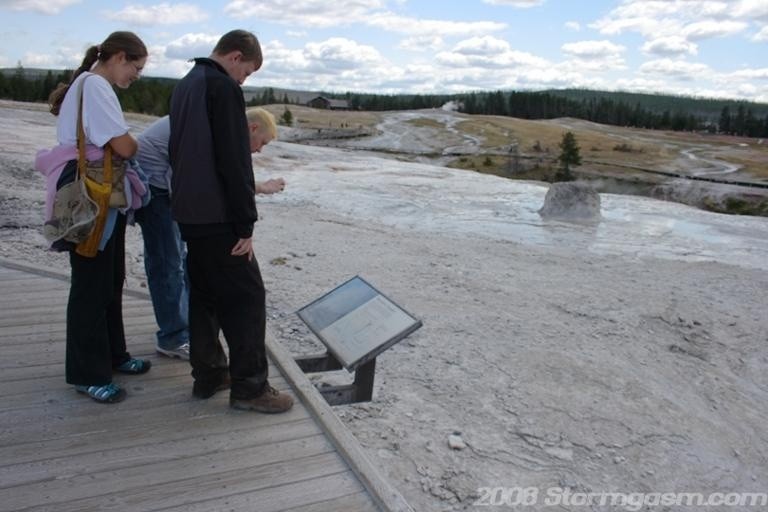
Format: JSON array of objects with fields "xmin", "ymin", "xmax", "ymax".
[
  {"xmin": 34, "ymin": 29, "xmax": 153, "ymax": 402},
  {"xmin": 132, "ymin": 106, "xmax": 287, "ymax": 361},
  {"xmin": 168, "ymin": 29, "xmax": 295, "ymax": 415}
]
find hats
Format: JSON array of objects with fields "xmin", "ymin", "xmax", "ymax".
[{"xmin": 42, "ymin": 181, "xmax": 102, "ymax": 252}]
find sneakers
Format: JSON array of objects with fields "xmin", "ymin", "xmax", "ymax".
[
  {"xmin": 193, "ymin": 366, "xmax": 294, "ymax": 413},
  {"xmin": 156, "ymin": 338, "xmax": 190, "ymax": 361}
]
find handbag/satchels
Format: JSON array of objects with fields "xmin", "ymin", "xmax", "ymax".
[
  {"xmin": 82, "ymin": 164, "xmax": 126, "ymax": 209},
  {"xmin": 71, "ymin": 176, "xmax": 112, "ymax": 260}
]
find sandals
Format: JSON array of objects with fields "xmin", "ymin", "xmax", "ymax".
[
  {"xmin": 75, "ymin": 381, "xmax": 127, "ymax": 404},
  {"xmin": 117, "ymin": 359, "xmax": 152, "ymax": 374}
]
